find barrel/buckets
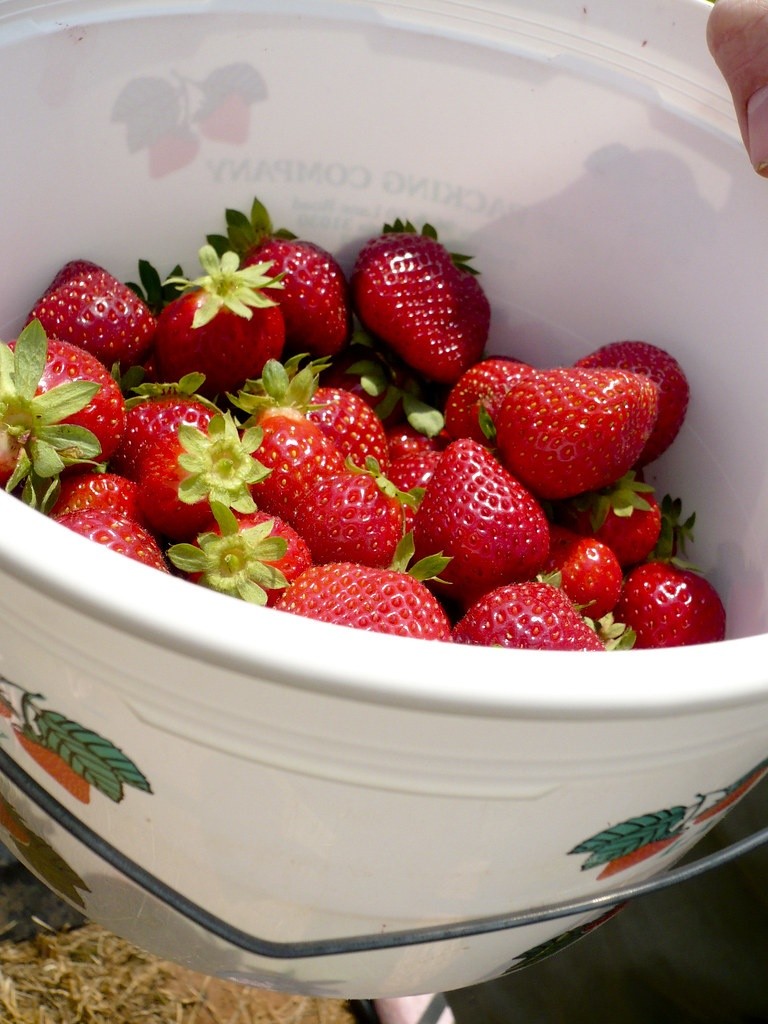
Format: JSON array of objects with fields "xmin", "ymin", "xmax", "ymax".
[{"xmin": 0, "ymin": 0, "xmax": 768, "ymax": 999}]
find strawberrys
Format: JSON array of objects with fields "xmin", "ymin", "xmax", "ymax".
[{"xmin": 0, "ymin": 232, "xmax": 726, "ymax": 651}]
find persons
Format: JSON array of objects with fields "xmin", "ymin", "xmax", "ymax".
[{"xmin": 707, "ymin": 0, "xmax": 768, "ymax": 178}]
